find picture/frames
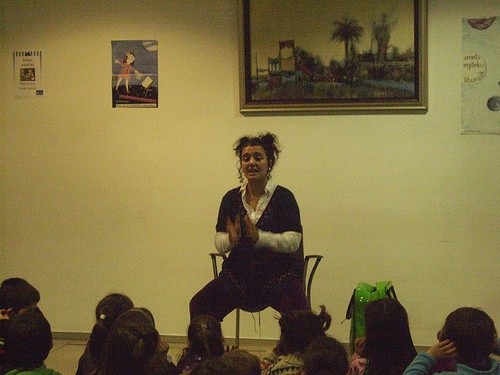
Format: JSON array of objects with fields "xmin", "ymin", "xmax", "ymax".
[{"xmin": 234, "ymin": 0, "xmax": 429, "ymax": 117}]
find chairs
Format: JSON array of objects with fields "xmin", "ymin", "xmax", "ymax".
[{"xmin": 208, "ymin": 225, "xmax": 323, "ymax": 346}]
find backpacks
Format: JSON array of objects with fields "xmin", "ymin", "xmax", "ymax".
[{"xmin": 345, "ymin": 280, "xmax": 398, "ymax": 354}]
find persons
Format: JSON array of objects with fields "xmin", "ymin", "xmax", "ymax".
[
  {"xmin": 75, "ymin": 294, "xmax": 500, "ymax": 375},
  {"xmin": 189, "ymin": 131, "xmax": 309, "ymax": 318},
  {"xmin": 0, "ymin": 278, "xmax": 64, "ymax": 375}
]
